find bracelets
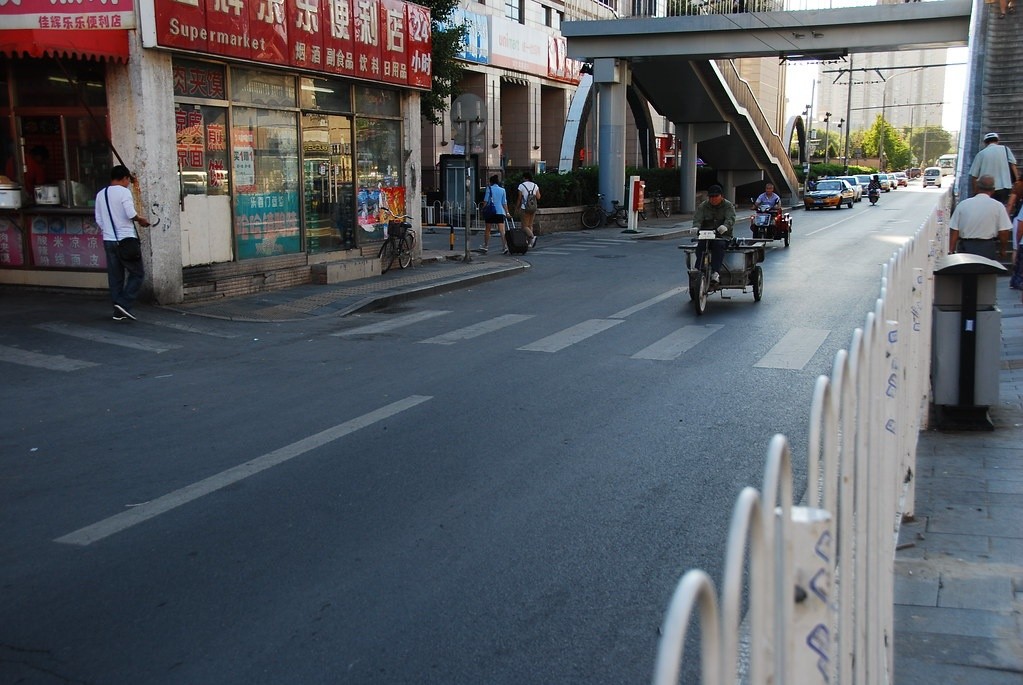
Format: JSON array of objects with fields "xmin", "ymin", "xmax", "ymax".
[{"xmin": 948, "ymin": 251, "xmax": 955, "ymax": 255}]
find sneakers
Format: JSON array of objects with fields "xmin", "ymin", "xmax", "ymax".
[
  {"xmin": 710, "ymin": 272, "xmax": 720, "ymax": 283},
  {"xmin": 112, "ymin": 311, "xmax": 128, "ymax": 320},
  {"xmin": 689, "ymin": 267, "xmax": 700, "ymax": 271},
  {"xmin": 480, "ymin": 245, "xmax": 488, "ymax": 251},
  {"xmin": 114, "ymin": 303, "xmax": 136, "ymax": 320},
  {"xmin": 501, "ymin": 247, "xmax": 508, "ymax": 254},
  {"xmin": 526, "ymin": 240, "xmax": 533, "ymax": 247},
  {"xmin": 531, "ymin": 236, "xmax": 537, "ymax": 247}
]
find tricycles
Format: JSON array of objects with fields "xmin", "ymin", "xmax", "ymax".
[
  {"xmin": 678, "ymin": 227, "xmax": 774, "ymax": 314},
  {"xmin": 749, "ymin": 198, "xmax": 794, "ymax": 246}
]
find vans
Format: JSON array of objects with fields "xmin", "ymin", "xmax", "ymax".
[
  {"xmin": 179, "ymin": 170, "xmax": 229, "ymax": 190},
  {"xmin": 922, "ymin": 167, "xmax": 942, "ymax": 188}
]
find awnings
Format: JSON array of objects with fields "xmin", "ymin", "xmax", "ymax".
[{"xmin": 0, "ymin": 0, "xmax": 133, "ymax": 65}]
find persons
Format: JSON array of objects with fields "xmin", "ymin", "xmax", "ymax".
[
  {"xmin": 477, "ymin": 174, "xmax": 513, "ymax": 254},
  {"xmin": 696, "ymin": 157, "xmax": 704, "ymax": 168},
  {"xmin": 751, "ymin": 184, "xmax": 782, "ymax": 239},
  {"xmin": 947, "ymin": 174, "xmax": 1013, "ymax": 262},
  {"xmin": 968, "ymin": 132, "xmax": 1020, "ymax": 209},
  {"xmin": 808, "ymin": 177, "xmax": 817, "ymax": 191},
  {"xmin": 1010, "ymin": 197, "xmax": 1023, "ymax": 269},
  {"xmin": 513, "ymin": 171, "xmax": 542, "ymax": 248},
  {"xmin": 1009, "ymin": 201, "xmax": 1023, "ymax": 293},
  {"xmin": 1004, "ymin": 168, "xmax": 1023, "ymax": 224},
  {"xmin": 690, "ymin": 184, "xmax": 737, "ymax": 284},
  {"xmin": 868, "ymin": 175, "xmax": 881, "ymax": 200},
  {"xmin": 998, "ymin": 0, "xmax": 1017, "ymax": 19},
  {"xmin": 4, "ymin": 143, "xmax": 49, "ymax": 194},
  {"xmin": 94, "ymin": 165, "xmax": 152, "ymax": 321},
  {"xmin": 579, "ymin": 146, "xmax": 586, "ymax": 167}
]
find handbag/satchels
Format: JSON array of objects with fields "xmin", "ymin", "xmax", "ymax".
[
  {"xmin": 115, "ymin": 241, "xmax": 140, "ymax": 269},
  {"xmin": 483, "ymin": 204, "xmax": 495, "ymax": 219},
  {"xmin": 1009, "ymin": 162, "xmax": 1016, "ymax": 182}
]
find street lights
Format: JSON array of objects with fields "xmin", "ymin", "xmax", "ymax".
[
  {"xmin": 879, "ymin": 67, "xmax": 928, "ymax": 173},
  {"xmin": 808, "ymin": 79, "xmax": 822, "ymax": 141},
  {"xmin": 909, "ymin": 105, "xmax": 940, "ymax": 168},
  {"xmin": 838, "ymin": 118, "xmax": 846, "ymax": 164},
  {"xmin": 802, "ymin": 104, "xmax": 811, "ymax": 195},
  {"xmin": 824, "ymin": 112, "xmax": 832, "ymax": 164}
]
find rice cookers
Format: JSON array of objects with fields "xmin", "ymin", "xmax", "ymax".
[
  {"xmin": 34, "ymin": 185, "xmax": 60, "ymax": 205},
  {"xmin": 0, "ymin": 181, "xmax": 23, "ymax": 210}
]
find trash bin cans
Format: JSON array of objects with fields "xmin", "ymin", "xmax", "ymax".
[{"xmin": 931, "ymin": 253, "xmax": 1008, "ymax": 435}]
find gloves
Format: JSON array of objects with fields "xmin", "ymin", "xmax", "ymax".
[
  {"xmin": 717, "ymin": 225, "xmax": 727, "ymax": 234},
  {"xmin": 689, "ymin": 227, "xmax": 699, "ymax": 236}
]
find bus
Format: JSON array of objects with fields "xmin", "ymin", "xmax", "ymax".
[{"xmin": 936, "ymin": 154, "xmax": 957, "ymax": 176}]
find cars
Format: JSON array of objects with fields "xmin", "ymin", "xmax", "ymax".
[
  {"xmin": 854, "ymin": 175, "xmax": 873, "ymax": 195},
  {"xmin": 804, "ymin": 179, "xmax": 855, "ymax": 210},
  {"xmin": 905, "ymin": 168, "xmax": 921, "ymax": 178},
  {"xmin": 887, "ymin": 174, "xmax": 897, "ymax": 190},
  {"xmin": 870, "ymin": 174, "xmax": 891, "ymax": 192},
  {"xmin": 894, "ymin": 172, "xmax": 908, "ymax": 187},
  {"xmin": 835, "ymin": 176, "xmax": 862, "ymax": 202}
]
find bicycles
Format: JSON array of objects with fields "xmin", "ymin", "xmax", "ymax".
[
  {"xmin": 648, "ymin": 190, "xmax": 671, "ymax": 220},
  {"xmin": 581, "ymin": 192, "xmax": 629, "ymax": 228},
  {"xmin": 378, "ymin": 206, "xmax": 415, "ymax": 274},
  {"xmin": 638, "ymin": 207, "xmax": 649, "ymax": 222}
]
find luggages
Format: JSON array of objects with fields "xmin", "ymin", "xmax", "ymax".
[{"xmin": 505, "ymin": 217, "xmax": 528, "ymax": 255}]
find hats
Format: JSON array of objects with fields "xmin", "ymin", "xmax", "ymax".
[
  {"xmin": 984, "ymin": 132, "xmax": 998, "ymax": 141},
  {"xmin": 707, "ymin": 185, "xmax": 723, "ymax": 196},
  {"xmin": 112, "ymin": 165, "xmax": 135, "ymax": 179}
]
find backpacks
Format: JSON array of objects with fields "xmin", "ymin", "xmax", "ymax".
[{"xmin": 521, "ymin": 182, "xmax": 538, "ymax": 213}]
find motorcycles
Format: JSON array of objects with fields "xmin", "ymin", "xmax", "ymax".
[{"xmin": 869, "ymin": 187, "xmax": 880, "ymax": 205}]
